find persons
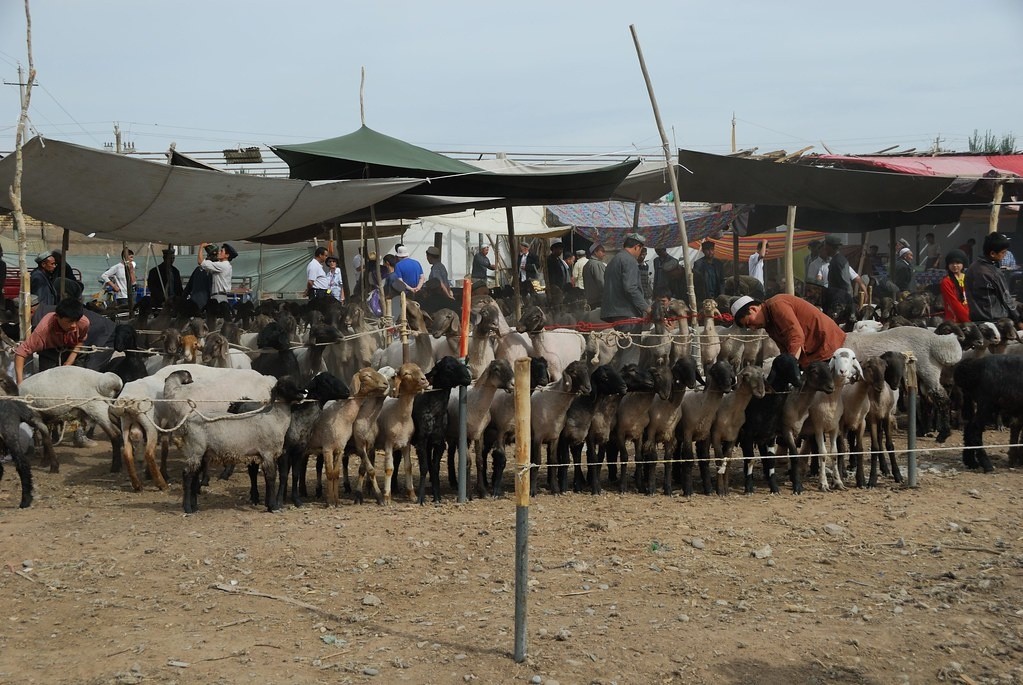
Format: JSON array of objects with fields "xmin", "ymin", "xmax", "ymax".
[
  {"xmin": 731, "ymin": 294, "xmax": 844, "ymax": 375},
  {"xmin": 14, "ymin": 298, "xmax": 98, "ymax": 447},
  {"xmin": 1, "ymin": 227, "xmax": 1023, "ymax": 330},
  {"xmin": 14, "ymin": 293, "xmax": 116, "ymax": 438}
]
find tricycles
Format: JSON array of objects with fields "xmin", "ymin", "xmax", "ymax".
[
  {"xmin": 3, "ymin": 267, "xmax": 82, "ymax": 300},
  {"xmin": 86, "ymin": 253, "xmax": 253, "ymax": 309}
]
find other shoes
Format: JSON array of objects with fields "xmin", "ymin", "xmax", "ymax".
[
  {"xmin": 73, "ymin": 430, "xmax": 99, "ymax": 448},
  {"xmin": 784, "ymin": 458, "xmax": 811, "ymax": 476},
  {"xmin": 51, "ymin": 420, "xmax": 67, "ymax": 446},
  {"xmin": 754, "ymin": 454, "xmax": 789, "ymax": 470}
]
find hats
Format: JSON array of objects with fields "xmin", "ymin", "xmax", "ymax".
[
  {"xmin": 900, "ymin": 248, "xmax": 910, "ymax": 257},
  {"xmin": 521, "ymin": 242, "xmax": 529, "ymax": 248},
  {"xmin": 472, "ymin": 280, "xmax": 486, "ymax": 290},
  {"xmin": 576, "ymin": 250, "xmax": 586, "ymax": 255},
  {"xmin": 325, "ymin": 256, "xmax": 338, "ymax": 267},
  {"xmin": 223, "ymin": 244, "xmax": 238, "ymax": 258},
  {"xmin": 427, "ymin": 246, "xmax": 439, "ymax": 255},
  {"xmin": 589, "ymin": 243, "xmax": 599, "ymax": 254},
  {"xmin": 162, "ymin": 249, "xmax": 175, "ymax": 254},
  {"xmin": 730, "ymin": 295, "xmax": 755, "ymax": 317},
  {"xmin": 945, "ymin": 248, "xmax": 968, "ymax": 264},
  {"xmin": 641, "ymin": 248, "xmax": 647, "ymax": 253},
  {"xmin": 481, "ymin": 244, "xmax": 489, "ymax": 250},
  {"xmin": 630, "ymin": 233, "xmax": 646, "ymax": 245},
  {"xmin": 898, "ymin": 239, "xmax": 911, "ymax": 248},
  {"xmin": 825, "ymin": 235, "xmax": 842, "ymax": 246},
  {"xmin": 397, "ymin": 246, "xmax": 409, "ymax": 257},
  {"xmin": 34, "ymin": 250, "xmax": 52, "ymax": 264}
]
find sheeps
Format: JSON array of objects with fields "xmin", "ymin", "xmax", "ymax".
[
  {"xmin": 103, "ymin": 295, "xmax": 1023, "ymax": 515},
  {"xmin": 0, "ymin": 331, "xmax": 125, "ymax": 509}
]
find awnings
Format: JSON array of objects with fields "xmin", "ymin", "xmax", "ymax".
[{"xmin": 0, "ymin": 126, "xmax": 1023, "ymax": 246}]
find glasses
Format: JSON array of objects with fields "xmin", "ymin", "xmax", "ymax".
[
  {"xmin": 322, "ymin": 254, "xmax": 327, "ymax": 257},
  {"xmin": 329, "ymin": 261, "xmax": 335, "ymax": 263}
]
